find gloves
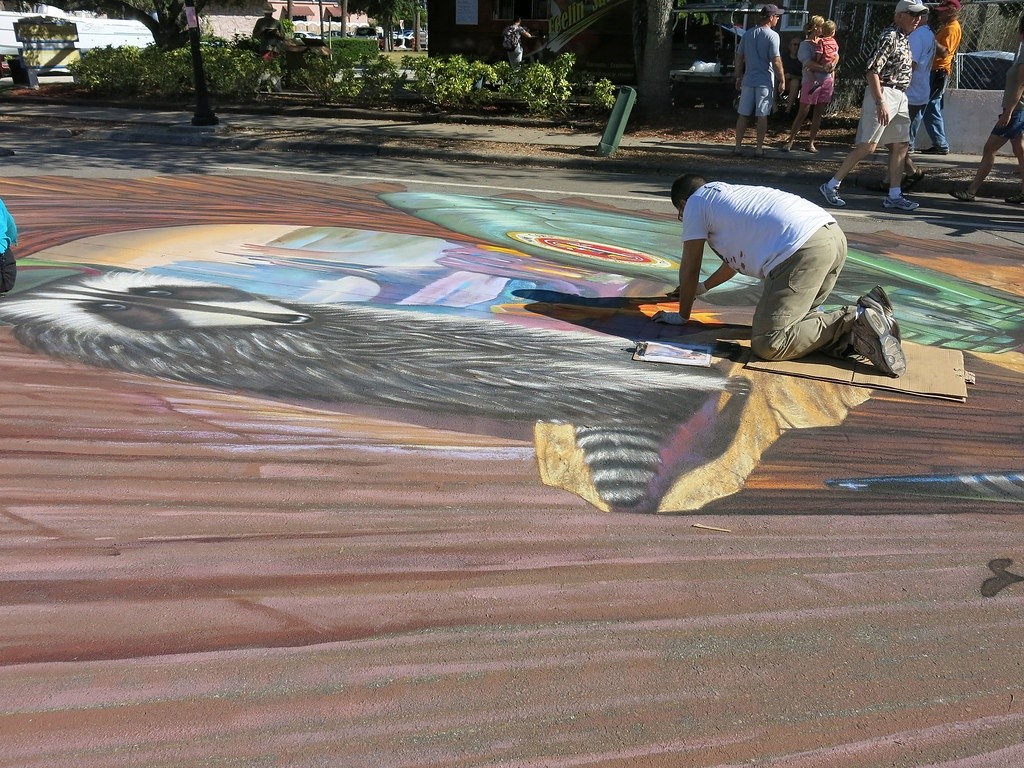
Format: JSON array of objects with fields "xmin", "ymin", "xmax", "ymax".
[
  {"xmin": 669, "ymin": 282, "xmax": 707, "ymax": 302},
  {"xmin": 650, "ymin": 310, "xmax": 690, "ymax": 326}
]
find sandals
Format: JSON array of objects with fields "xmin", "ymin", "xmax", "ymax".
[
  {"xmin": 1005, "ymin": 193, "xmax": 1024, "ymax": 204},
  {"xmin": 782, "ymin": 145, "xmax": 790, "ymax": 152},
  {"xmin": 802, "ymin": 147, "xmax": 820, "ymax": 153},
  {"xmin": 900, "ymin": 166, "xmax": 924, "ymax": 192},
  {"xmin": 949, "ymin": 189, "xmax": 977, "ymax": 202}
]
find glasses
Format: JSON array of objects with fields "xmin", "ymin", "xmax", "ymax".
[
  {"xmin": 907, "ymin": 10, "xmax": 925, "ymax": 16},
  {"xmin": 677, "ymin": 204, "xmax": 684, "ymax": 222}
]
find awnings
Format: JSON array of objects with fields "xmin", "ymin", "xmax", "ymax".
[
  {"xmin": 283, "ymin": 6, "xmax": 314, "ymax": 17},
  {"xmin": 325, "ymin": 7, "xmax": 349, "ymax": 17}
]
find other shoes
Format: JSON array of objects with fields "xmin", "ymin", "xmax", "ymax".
[
  {"xmin": 807, "ymin": 80, "xmax": 822, "ymax": 95},
  {"xmin": 907, "ymin": 148, "xmax": 915, "ymax": 154},
  {"xmin": 921, "ymin": 145, "xmax": 949, "ymax": 155}
]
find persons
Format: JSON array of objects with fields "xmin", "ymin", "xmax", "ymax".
[
  {"xmin": 0, "ymin": 198, "xmax": 20, "ymax": 292},
  {"xmin": 949, "ymin": 42, "xmax": 1023, "ymax": 201},
  {"xmin": 782, "ymin": 14, "xmax": 840, "ymax": 153},
  {"xmin": 882, "ymin": 0, "xmax": 963, "ymax": 188},
  {"xmin": 502, "ymin": 16, "xmax": 531, "ymax": 71},
  {"xmin": 732, "ymin": 3, "xmax": 786, "ymax": 157},
  {"xmin": 819, "ymin": 0, "xmax": 929, "ymax": 210},
  {"xmin": 649, "ymin": 168, "xmax": 907, "ymax": 378},
  {"xmin": 772, "ymin": 34, "xmax": 803, "ymax": 118},
  {"xmin": 252, "ymin": 3, "xmax": 283, "ymax": 38}
]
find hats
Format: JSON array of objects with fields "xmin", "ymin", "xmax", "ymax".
[
  {"xmin": 934, "ymin": 0, "xmax": 962, "ymax": 13},
  {"xmin": 761, "ymin": 4, "xmax": 785, "ymax": 17},
  {"xmin": 259, "ymin": 2, "xmax": 277, "ymax": 13},
  {"xmin": 894, "ymin": 0, "xmax": 929, "ymax": 15}
]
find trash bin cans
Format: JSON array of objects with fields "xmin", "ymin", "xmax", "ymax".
[
  {"xmin": 378, "ymin": 37, "xmax": 384, "ymax": 50},
  {"xmin": 958, "ymin": 51, "xmax": 1016, "ymax": 90},
  {"xmin": 283, "ymin": 38, "xmax": 336, "ymax": 69}
]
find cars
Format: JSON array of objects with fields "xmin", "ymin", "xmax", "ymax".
[{"xmin": 293, "ymin": 27, "xmax": 428, "ymax": 51}]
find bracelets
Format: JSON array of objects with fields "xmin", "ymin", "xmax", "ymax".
[
  {"xmin": 736, "ymin": 76, "xmax": 742, "ymax": 81},
  {"xmin": 876, "ymin": 99, "xmax": 885, "ymax": 105}
]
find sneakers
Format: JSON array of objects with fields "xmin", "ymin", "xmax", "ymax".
[
  {"xmin": 883, "ymin": 192, "xmax": 919, "ymax": 210},
  {"xmin": 819, "ymin": 182, "xmax": 846, "ymax": 207},
  {"xmin": 857, "ymin": 285, "xmax": 901, "ymax": 348},
  {"xmin": 851, "ymin": 307, "xmax": 907, "ymax": 378}
]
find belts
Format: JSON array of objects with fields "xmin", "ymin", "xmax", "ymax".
[{"xmin": 881, "ymin": 83, "xmax": 906, "ymax": 92}]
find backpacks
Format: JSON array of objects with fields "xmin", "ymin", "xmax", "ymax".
[{"xmin": 503, "ymin": 26, "xmax": 524, "ymax": 52}]
